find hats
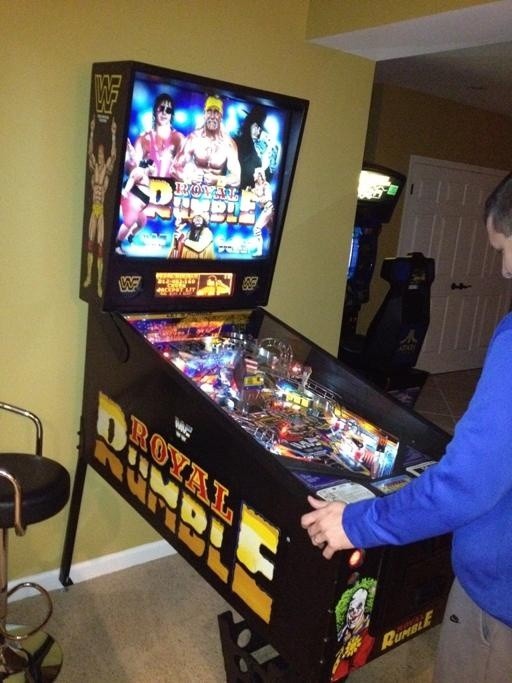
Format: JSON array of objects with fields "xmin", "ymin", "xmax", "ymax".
[
  {"xmin": 205, "ymin": 96, "xmax": 224, "ymax": 117},
  {"xmin": 240, "ymin": 104, "xmax": 268, "ymax": 134}
]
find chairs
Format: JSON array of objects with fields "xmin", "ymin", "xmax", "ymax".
[{"xmin": 344, "ymin": 249, "xmax": 438, "ymax": 410}]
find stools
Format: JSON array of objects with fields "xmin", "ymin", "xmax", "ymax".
[{"xmin": 0, "ymin": 400, "xmax": 72, "ymax": 683}]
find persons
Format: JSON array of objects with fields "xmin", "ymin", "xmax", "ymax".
[
  {"xmin": 240, "ymin": 161, "xmax": 277, "ymax": 261},
  {"xmin": 113, "ymin": 156, "xmax": 158, "ymax": 256},
  {"xmin": 232, "ymin": 104, "xmax": 284, "ymax": 189},
  {"xmin": 297, "ymin": 168, "xmax": 512, "ymax": 679},
  {"xmin": 167, "ymin": 94, "xmax": 244, "ymax": 192},
  {"xmin": 170, "ymin": 205, "xmax": 219, "ymax": 260},
  {"xmin": 123, "ymin": 94, "xmax": 187, "ymax": 221}
]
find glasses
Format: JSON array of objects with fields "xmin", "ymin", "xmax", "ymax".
[{"xmin": 155, "ymin": 105, "xmax": 172, "ymax": 114}]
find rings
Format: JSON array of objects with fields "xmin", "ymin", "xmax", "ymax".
[{"xmin": 314, "ymin": 536, "xmax": 321, "ymax": 545}]
